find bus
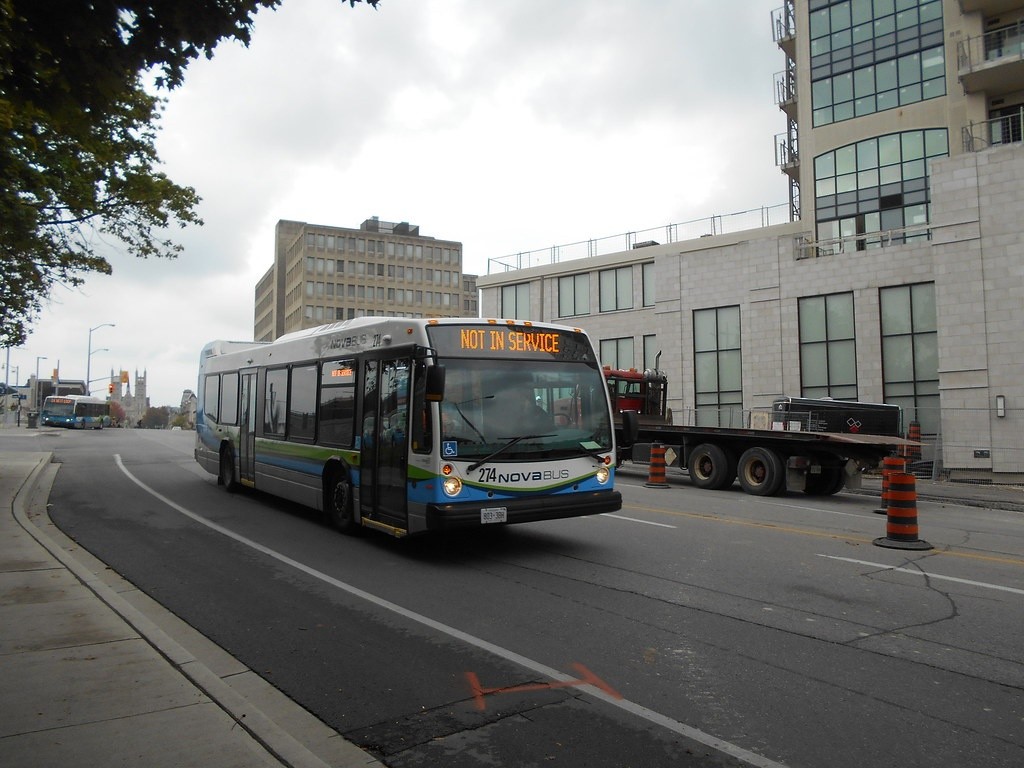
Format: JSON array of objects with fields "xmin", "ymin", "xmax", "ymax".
[
  {"xmin": 40, "ymin": 395, "xmax": 112, "ymax": 430},
  {"xmin": 194, "ymin": 316, "xmax": 624, "ymax": 546}
]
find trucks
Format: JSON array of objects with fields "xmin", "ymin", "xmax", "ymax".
[{"xmin": 550, "ymin": 349, "xmax": 931, "ymax": 497}]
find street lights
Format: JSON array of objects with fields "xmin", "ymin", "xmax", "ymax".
[
  {"xmin": 85, "ymin": 323, "xmax": 115, "ymax": 394},
  {"xmin": 2, "ymin": 363, "xmax": 20, "ymax": 427},
  {"xmin": 35, "ymin": 356, "xmax": 48, "ymax": 412}
]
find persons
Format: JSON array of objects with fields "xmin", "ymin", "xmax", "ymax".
[
  {"xmin": 519, "ymin": 390, "xmax": 547, "ymax": 419},
  {"xmin": 124, "ymin": 418, "xmax": 129, "ymax": 429},
  {"xmin": 139, "ymin": 420, "xmax": 142, "ymax": 429},
  {"xmin": 112, "ymin": 417, "xmax": 116, "ymax": 428},
  {"xmin": 117, "ymin": 420, "xmax": 120, "ymax": 428}
]
